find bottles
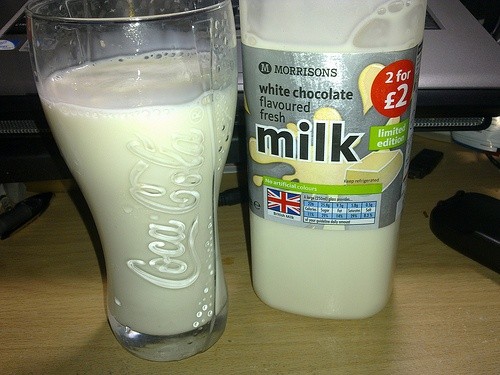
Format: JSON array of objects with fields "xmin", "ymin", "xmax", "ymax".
[{"xmin": 239, "ymin": 1, "xmax": 427, "ymax": 321}]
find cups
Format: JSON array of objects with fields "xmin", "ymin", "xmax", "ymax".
[{"xmin": 24, "ymin": 0, "xmax": 238, "ymax": 363}]
